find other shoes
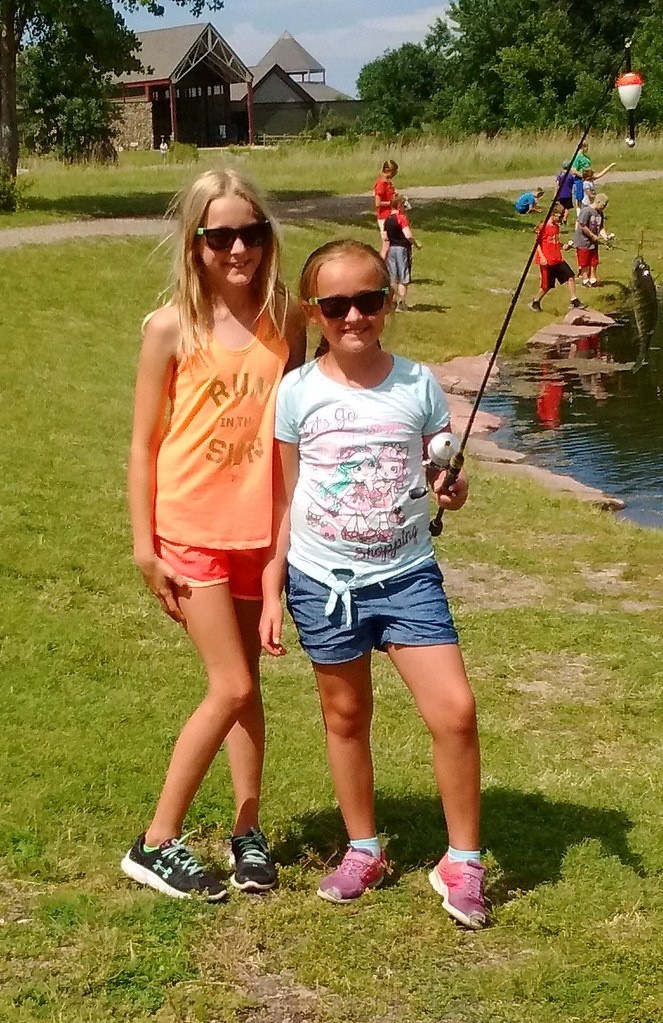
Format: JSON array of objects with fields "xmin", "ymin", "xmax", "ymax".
[
  {"xmin": 392, "ymin": 302, "xmax": 410, "ymax": 312},
  {"xmin": 581, "ymin": 280, "xmax": 604, "ymax": 288}
]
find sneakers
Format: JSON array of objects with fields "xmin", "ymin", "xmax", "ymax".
[
  {"xmin": 229, "ymin": 831, "xmax": 277, "ymax": 890},
  {"xmin": 528, "ymin": 298, "xmax": 542, "ymax": 312},
  {"xmin": 428, "ymin": 852, "xmax": 489, "ymax": 929},
  {"xmin": 121, "ymin": 831, "xmax": 227, "ymax": 901},
  {"xmin": 317, "ymin": 848, "xmax": 387, "ymax": 904},
  {"xmin": 569, "ymin": 297, "xmax": 589, "ymax": 309}
]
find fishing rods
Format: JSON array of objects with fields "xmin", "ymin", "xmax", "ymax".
[
  {"xmin": 410, "ymin": 28, "xmax": 642, "ymax": 536},
  {"xmin": 598, "ymin": 241, "xmax": 626, "ymax": 253}
]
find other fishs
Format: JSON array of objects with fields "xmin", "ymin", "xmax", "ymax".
[{"xmin": 630, "ymin": 256, "xmax": 658, "ymax": 375}]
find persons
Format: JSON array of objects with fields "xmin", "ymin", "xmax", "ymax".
[
  {"xmin": 528, "ymin": 202, "xmax": 588, "ymax": 312},
  {"xmin": 375, "ymin": 159, "xmax": 424, "ymax": 312},
  {"xmin": 575, "ymin": 193, "xmax": 610, "ymax": 286},
  {"xmin": 260, "ymin": 240, "xmax": 485, "ymax": 930},
  {"xmin": 121, "ymin": 168, "xmax": 307, "ymax": 901},
  {"xmin": 160, "ymin": 139, "xmax": 168, "ymax": 164},
  {"xmin": 516, "ymin": 140, "xmax": 617, "ymax": 225}
]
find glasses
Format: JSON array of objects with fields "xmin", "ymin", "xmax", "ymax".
[
  {"xmin": 555, "ymin": 213, "xmax": 564, "ymax": 218},
  {"xmin": 196, "ymin": 219, "xmax": 269, "ymax": 250},
  {"xmin": 306, "ymin": 287, "xmax": 390, "ymax": 319}
]
